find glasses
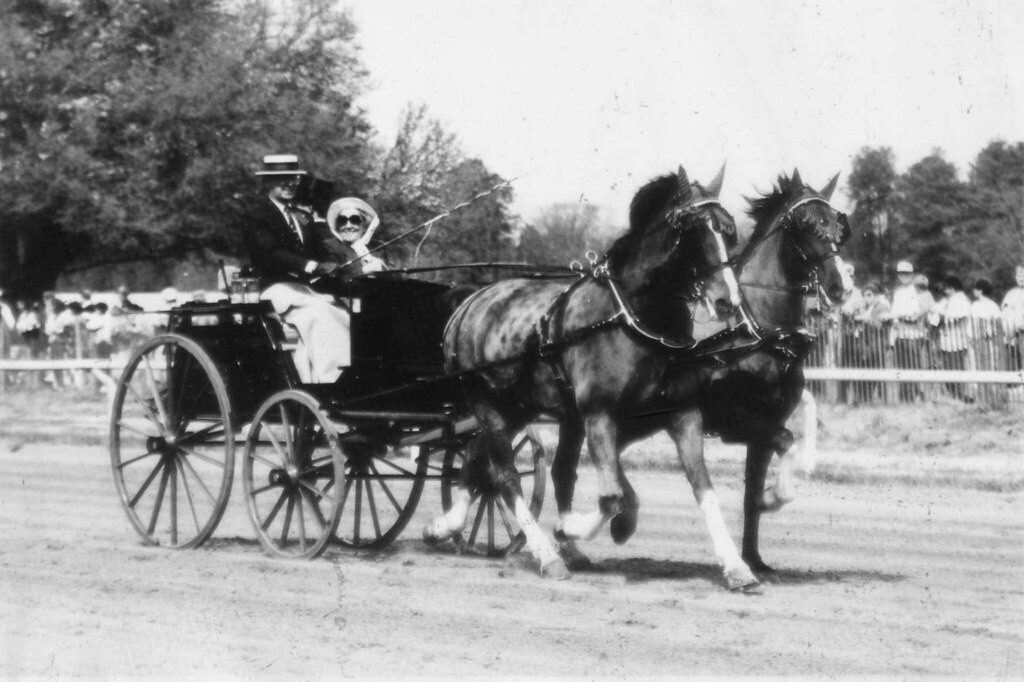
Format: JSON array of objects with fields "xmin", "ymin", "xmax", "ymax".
[
  {"xmin": 274, "ymin": 175, "xmax": 301, "ymax": 186},
  {"xmin": 336, "ymin": 215, "xmax": 363, "ymax": 226}
]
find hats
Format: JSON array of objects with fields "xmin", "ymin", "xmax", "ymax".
[
  {"xmin": 328, "ymin": 196, "xmax": 380, "ymax": 243},
  {"xmin": 253, "ymin": 155, "xmax": 306, "ymax": 175}
]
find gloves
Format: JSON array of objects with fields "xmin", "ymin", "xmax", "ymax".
[{"xmin": 315, "ymin": 263, "xmax": 341, "ymax": 278}]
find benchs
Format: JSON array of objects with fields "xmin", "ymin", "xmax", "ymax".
[{"xmin": 238, "ymin": 246, "xmax": 453, "ymax": 404}]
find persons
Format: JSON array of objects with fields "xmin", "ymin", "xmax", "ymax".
[
  {"xmin": 242, "ymin": 153, "xmax": 353, "ymax": 384},
  {"xmin": 0, "ymin": 259, "xmax": 240, "ymax": 401},
  {"xmin": 322, "ymin": 196, "xmax": 390, "ymax": 312},
  {"xmin": 805, "ymin": 258, "xmax": 1024, "ymax": 407}
]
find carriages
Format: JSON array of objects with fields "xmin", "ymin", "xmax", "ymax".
[{"xmin": 107, "ymin": 158, "xmax": 855, "ymax": 593}]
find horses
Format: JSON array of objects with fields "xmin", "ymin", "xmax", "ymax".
[{"xmin": 417, "ymin": 161, "xmax": 851, "ymax": 590}]
